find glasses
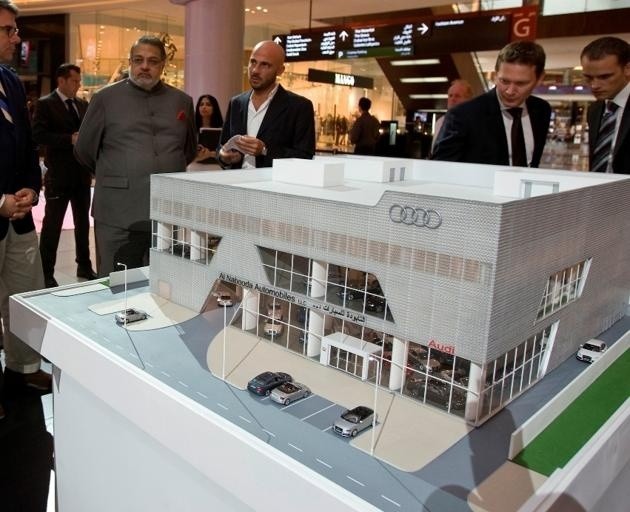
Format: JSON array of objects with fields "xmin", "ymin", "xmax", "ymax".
[{"xmin": 0, "ymin": 26, "xmax": 19, "ymax": 38}]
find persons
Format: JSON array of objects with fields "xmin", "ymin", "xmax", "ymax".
[
  {"xmin": 432, "ymin": 41, "xmax": 551, "ymax": 167},
  {"xmin": 74, "ymin": 33, "xmax": 197, "ymax": 279},
  {"xmin": 579, "ymin": 37, "xmax": 630, "ymax": 175},
  {"xmin": 217, "ymin": 39, "xmax": 317, "ymax": 174},
  {"xmin": 426, "ymin": 80, "xmax": 475, "ymax": 158},
  {"xmin": 313, "ymin": 111, "xmax": 359, "ymax": 147},
  {"xmin": 0, "ymin": 0, "xmax": 51, "ymax": 419},
  {"xmin": 189, "ymin": 93, "xmax": 228, "ymax": 166},
  {"xmin": 415, "ymin": 116, "xmax": 424, "ymax": 132},
  {"xmin": 349, "ymin": 98, "xmax": 381, "ymax": 156},
  {"xmin": 31, "ymin": 62, "xmax": 102, "ymax": 291}
]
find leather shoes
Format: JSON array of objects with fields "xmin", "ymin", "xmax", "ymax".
[{"xmin": 77, "ymin": 268, "xmax": 97, "ymax": 279}]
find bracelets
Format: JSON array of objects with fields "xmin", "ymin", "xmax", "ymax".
[{"xmin": 0, "ymin": 193, "xmax": 6, "ymax": 208}]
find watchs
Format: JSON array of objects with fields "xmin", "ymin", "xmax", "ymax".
[{"xmin": 262, "ymin": 145, "xmax": 269, "ymax": 157}]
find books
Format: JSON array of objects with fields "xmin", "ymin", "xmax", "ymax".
[{"xmin": 199, "ymin": 127, "xmax": 223, "ymax": 155}]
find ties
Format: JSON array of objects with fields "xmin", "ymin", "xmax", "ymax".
[
  {"xmin": 590, "ymin": 100, "xmax": 620, "ymax": 173},
  {"xmin": 506, "ymin": 106, "xmax": 528, "ymax": 167},
  {"xmin": 65, "ymin": 99, "xmax": 78, "ymax": 119}
]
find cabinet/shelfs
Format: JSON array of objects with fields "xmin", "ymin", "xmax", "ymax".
[{"xmin": 6, "ymin": 266, "xmax": 630, "ymax": 512}]
